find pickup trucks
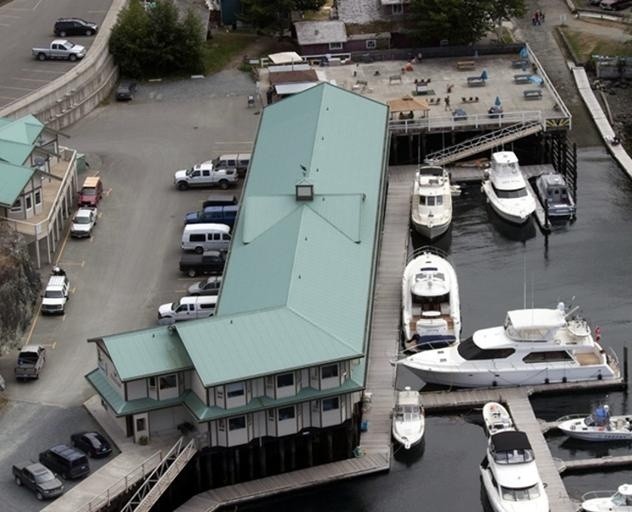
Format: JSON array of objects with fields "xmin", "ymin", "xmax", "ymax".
[
  {"xmin": 176, "ymin": 249, "xmax": 226, "ymax": 277},
  {"xmin": 11, "ymin": 457, "xmax": 66, "ymax": 501},
  {"xmin": 13, "ymin": 343, "xmax": 49, "ymax": 384},
  {"xmin": 68, "ymin": 205, "xmax": 99, "ymax": 242},
  {"xmin": 156, "ymin": 294, "xmax": 218, "ymax": 320},
  {"xmin": 172, "ymin": 158, "xmax": 239, "ymax": 192},
  {"xmin": 181, "ymin": 205, "xmax": 239, "ymax": 228},
  {"xmin": 32, "ymin": 39, "xmax": 86, "ymax": 63}
]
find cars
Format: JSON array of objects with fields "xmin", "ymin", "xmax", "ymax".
[
  {"xmin": 186, "ymin": 276, "xmax": 223, "ymax": 296},
  {"xmin": 68, "ymin": 428, "xmax": 113, "ymax": 460},
  {"xmin": 201, "ymin": 194, "xmax": 237, "ymax": 209}
]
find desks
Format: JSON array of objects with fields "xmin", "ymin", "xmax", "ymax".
[{"xmin": 391, "ymin": 59, "xmax": 542, "ymax": 119}]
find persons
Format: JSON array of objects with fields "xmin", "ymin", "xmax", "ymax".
[
  {"xmin": 532, "ymin": 10, "xmax": 545, "ymax": 26},
  {"xmin": 444, "ymin": 96, "xmax": 452, "ymax": 111}
]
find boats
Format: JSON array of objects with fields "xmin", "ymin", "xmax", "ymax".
[
  {"xmin": 478, "ymin": 149, "xmax": 538, "ymax": 228},
  {"xmin": 475, "ymin": 427, "xmax": 551, "ymax": 512},
  {"xmin": 578, "ymin": 482, "xmax": 632, "ymax": 512},
  {"xmin": 480, "ymin": 203, "xmax": 538, "ymax": 249},
  {"xmin": 391, "ymin": 438, "xmax": 426, "ymax": 470},
  {"xmin": 556, "ymin": 413, "xmax": 632, "ymax": 440},
  {"xmin": 387, "ymin": 384, "xmax": 427, "ymax": 451},
  {"xmin": 535, "ymin": 169, "xmax": 578, "ymax": 220},
  {"xmin": 396, "ymin": 245, "xmax": 463, "ymax": 353},
  {"xmin": 555, "ymin": 435, "xmax": 632, "ymax": 458},
  {"xmin": 480, "ymin": 400, "xmax": 515, "ymax": 434},
  {"xmin": 409, "ymin": 228, "xmax": 453, "ymax": 258},
  {"xmin": 410, "ymin": 162, "xmax": 460, "ymax": 238},
  {"xmin": 385, "ymin": 295, "xmax": 622, "ymax": 389}
]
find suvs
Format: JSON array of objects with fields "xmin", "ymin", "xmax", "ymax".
[
  {"xmin": 39, "ymin": 273, "xmax": 72, "ymax": 317},
  {"xmin": 52, "ymin": 16, "xmax": 99, "ymax": 38}
]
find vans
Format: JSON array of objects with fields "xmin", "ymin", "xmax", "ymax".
[
  {"xmin": 76, "ymin": 175, "xmax": 104, "ymax": 207},
  {"xmin": 114, "ymin": 77, "xmax": 138, "ymax": 103},
  {"xmin": 181, "ymin": 224, "xmax": 232, "ymax": 254},
  {"xmin": 209, "ymin": 153, "xmax": 252, "ymax": 173},
  {"xmin": 37, "ymin": 443, "xmax": 92, "ymax": 481}
]
